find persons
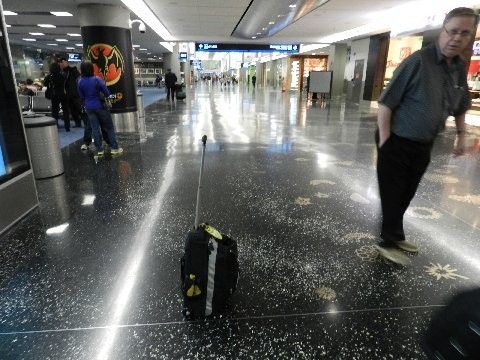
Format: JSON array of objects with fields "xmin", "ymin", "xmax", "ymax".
[
  {"xmin": 43, "ymin": 63, "xmax": 70, "ymax": 134},
  {"xmin": 154, "ymin": 74, "xmax": 161, "ymax": 85},
  {"xmin": 165, "ymin": 68, "xmax": 178, "ymax": 100},
  {"xmin": 374, "ymin": 6, "xmax": 479, "ymax": 252},
  {"xmin": 81, "ymin": 104, "xmax": 115, "ymax": 150},
  {"xmin": 77, "ymin": 61, "xmax": 123, "ymax": 155},
  {"xmin": 18, "ymin": 75, "xmax": 45, "ymax": 96},
  {"xmin": 200, "ymin": 73, "xmax": 257, "ymax": 87},
  {"xmin": 59, "ymin": 58, "xmax": 88, "ymax": 126}
]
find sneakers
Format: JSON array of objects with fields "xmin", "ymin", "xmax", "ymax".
[
  {"xmin": 66, "ymin": 128, "xmax": 70, "ymax": 131},
  {"xmin": 377, "ymin": 244, "xmax": 409, "ymax": 264},
  {"xmin": 98, "ymin": 150, "xmax": 104, "ymax": 155},
  {"xmin": 73, "ymin": 125, "xmax": 82, "ymax": 127},
  {"xmin": 110, "ymin": 148, "xmax": 123, "ymax": 155},
  {"xmin": 397, "ymin": 241, "xmax": 420, "ymax": 253},
  {"xmin": 81, "ymin": 144, "xmax": 88, "ymax": 150}
]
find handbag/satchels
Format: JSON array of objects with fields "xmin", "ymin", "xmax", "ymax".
[
  {"xmin": 45, "ymin": 75, "xmax": 55, "ymax": 99},
  {"xmin": 80, "ymin": 106, "xmax": 89, "ymax": 126},
  {"xmin": 95, "ymin": 76, "xmax": 113, "ymax": 111}
]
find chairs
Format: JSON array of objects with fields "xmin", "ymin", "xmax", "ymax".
[
  {"xmin": 19, "ymin": 96, "xmax": 31, "ymax": 110},
  {"xmin": 32, "ymin": 96, "xmax": 50, "ymax": 111},
  {"xmin": 36, "ymin": 91, "xmax": 45, "ymax": 96}
]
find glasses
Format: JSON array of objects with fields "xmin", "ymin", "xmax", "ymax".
[{"xmin": 443, "ymin": 25, "xmax": 475, "ymax": 38}]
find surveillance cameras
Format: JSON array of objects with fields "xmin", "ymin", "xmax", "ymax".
[{"xmin": 138, "ymin": 23, "xmax": 146, "ymax": 33}]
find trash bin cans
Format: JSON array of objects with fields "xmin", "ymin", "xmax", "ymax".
[
  {"xmin": 32, "ymin": 180, "xmax": 74, "ymax": 227},
  {"xmin": 136, "ymin": 91, "xmax": 147, "ymax": 141},
  {"xmin": 23, "ymin": 115, "xmax": 64, "ymax": 179}
]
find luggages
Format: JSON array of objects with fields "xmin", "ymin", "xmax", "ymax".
[
  {"xmin": 181, "ymin": 135, "xmax": 241, "ymax": 317},
  {"xmin": 176, "ymin": 84, "xmax": 186, "ymax": 100}
]
